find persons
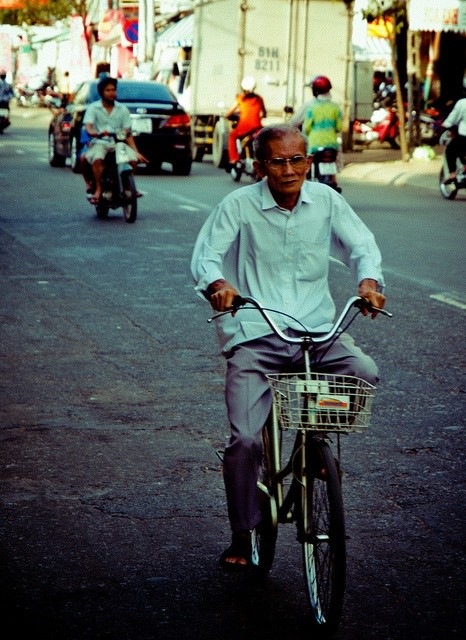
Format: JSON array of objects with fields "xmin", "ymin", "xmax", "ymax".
[
  {"xmin": 302, "ymin": 76, "xmax": 342, "ymax": 156},
  {"xmin": 0, "ymin": 69, "xmax": 15, "ymax": 102},
  {"xmin": 190, "ymin": 124, "xmax": 386, "ymax": 570},
  {"xmin": 60, "ymin": 71, "xmax": 69, "ymax": 108},
  {"xmin": 83, "ymin": 77, "xmax": 148, "ymax": 204},
  {"xmin": 287, "ymin": 82, "xmax": 337, "ymax": 133},
  {"xmin": 46, "ymin": 82, "xmax": 52, "ymax": 95},
  {"xmin": 78, "ymin": 83, "xmax": 100, "ymax": 193},
  {"xmin": 442, "ymin": 76, "xmax": 466, "ymax": 185},
  {"xmin": 53, "ymin": 82, "xmax": 60, "ymax": 96},
  {"xmin": 224, "ymin": 77, "xmax": 267, "ymax": 164}
]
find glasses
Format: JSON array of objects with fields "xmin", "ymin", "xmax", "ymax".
[{"xmin": 263, "ymin": 156, "xmax": 309, "ymax": 170}]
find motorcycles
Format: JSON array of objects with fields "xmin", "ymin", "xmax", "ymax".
[
  {"xmin": 95, "ymin": 129, "xmax": 137, "ymax": 223},
  {"xmin": 230, "ymin": 111, "xmax": 263, "ymax": 183},
  {"xmin": 284, "ymin": 106, "xmax": 342, "ymax": 194},
  {"xmin": 0, "ymin": 101, "xmax": 11, "ymax": 135},
  {"xmin": 354, "ymin": 97, "xmax": 412, "ymax": 151},
  {"xmin": 439, "ymin": 124, "xmax": 466, "ymax": 201}
]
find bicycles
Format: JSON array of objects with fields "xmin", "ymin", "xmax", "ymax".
[{"xmin": 207, "ymin": 296, "xmax": 392, "ymax": 632}]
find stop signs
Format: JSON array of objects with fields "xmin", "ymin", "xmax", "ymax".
[{"xmin": 124, "ymin": 19, "xmax": 138, "ymax": 44}]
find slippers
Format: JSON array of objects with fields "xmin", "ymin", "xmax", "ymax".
[
  {"xmin": 90, "ymin": 197, "xmax": 99, "ymax": 204},
  {"xmin": 128, "ymin": 191, "xmax": 142, "ymax": 199},
  {"xmin": 220, "ymin": 546, "xmax": 252, "ymax": 573},
  {"xmin": 315, "ymin": 458, "xmax": 341, "ymax": 482}
]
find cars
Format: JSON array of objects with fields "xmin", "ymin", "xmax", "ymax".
[{"xmin": 48, "ymin": 79, "xmax": 193, "ymax": 175}]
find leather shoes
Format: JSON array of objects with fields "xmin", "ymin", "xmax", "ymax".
[
  {"xmin": 444, "ymin": 178, "xmax": 457, "ymax": 185},
  {"xmin": 86, "ymin": 186, "xmax": 95, "ymax": 193},
  {"xmin": 462, "ymin": 171, "xmax": 466, "ymax": 175}
]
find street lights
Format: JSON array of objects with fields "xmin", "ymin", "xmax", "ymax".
[{"xmin": 363, "ymin": 0, "xmax": 412, "ymax": 140}]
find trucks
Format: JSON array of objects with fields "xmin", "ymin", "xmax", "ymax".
[{"xmin": 171, "ymin": 0, "xmax": 354, "ymax": 170}]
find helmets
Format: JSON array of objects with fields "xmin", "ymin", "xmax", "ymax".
[
  {"xmin": 312, "ymin": 76, "xmax": 332, "ymax": 97},
  {"xmin": 97, "ymin": 77, "xmax": 117, "ymax": 104},
  {"xmin": 0, "ymin": 68, "xmax": 6, "ymax": 75},
  {"xmin": 463, "ymin": 75, "xmax": 466, "ymax": 87},
  {"xmin": 242, "ymin": 77, "xmax": 256, "ymax": 92}
]
